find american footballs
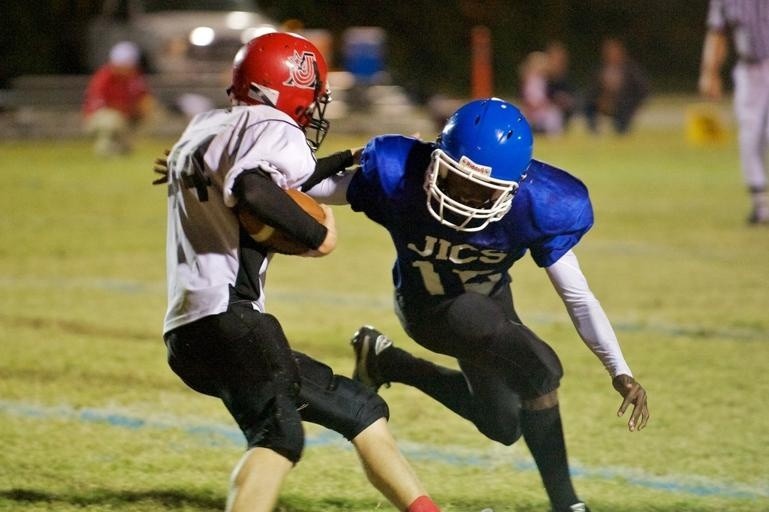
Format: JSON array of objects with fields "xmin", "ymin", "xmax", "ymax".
[{"xmin": 239, "ymin": 189, "xmax": 327, "ymax": 254}]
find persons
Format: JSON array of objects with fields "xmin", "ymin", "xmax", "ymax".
[
  {"xmin": 149, "ymin": 95, "xmax": 651, "ymax": 512},
  {"xmin": 518, "ymin": 33, "xmax": 646, "ymax": 136},
  {"xmin": 698, "ymin": 0, "xmax": 768, "ymax": 224},
  {"xmin": 162, "ymin": 32, "xmax": 442, "ymax": 512},
  {"xmin": 77, "ymin": 42, "xmax": 161, "ymax": 155}
]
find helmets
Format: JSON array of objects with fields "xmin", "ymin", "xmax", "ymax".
[
  {"xmin": 232, "ymin": 32, "xmax": 332, "ymax": 151},
  {"xmin": 424, "ymin": 97, "xmax": 533, "ymax": 233}
]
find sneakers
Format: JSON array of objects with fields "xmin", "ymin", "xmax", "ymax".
[{"xmin": 352, "ymin": 326, "xmax": 393, "ymax": 394}]
